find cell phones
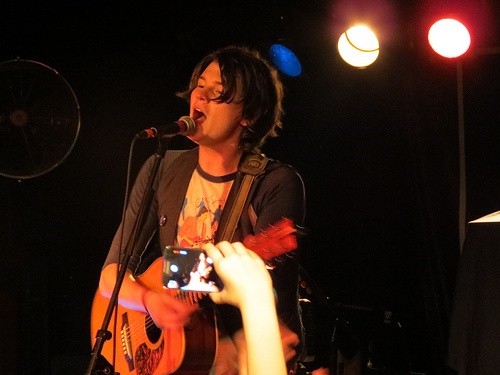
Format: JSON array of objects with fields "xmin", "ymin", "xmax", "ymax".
[{"xmin": 162, "ymin": 245, "xmax": 224, "ymax": 293}]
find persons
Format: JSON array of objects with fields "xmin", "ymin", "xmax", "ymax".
[
  {"xmin": 91, "ymin": 47, "xmax": 305, "ymax": 375},
  {"xmin": 203, "ymin": 241, "xmax": 288, "ymax": 375},
  {"xmin": 190, "ymin": 253, "xmax": 209, "ymax": 283}
]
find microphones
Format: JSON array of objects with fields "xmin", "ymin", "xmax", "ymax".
[{"xmin": 136, "ymin": 116, "xmax": 196, "ymax": 139}]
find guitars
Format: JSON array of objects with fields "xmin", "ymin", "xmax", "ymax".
[{"xmin": 83, "ymin": 217, "xmax": 301, "ymax": 375}]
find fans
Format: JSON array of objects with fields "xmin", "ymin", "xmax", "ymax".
[{"xmin": 0, "ymin": 59, "xmax": 81, "ymax": 179}]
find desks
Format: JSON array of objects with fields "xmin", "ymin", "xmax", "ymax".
[{"xmin": 448, "ymin": 210, "xmax": 500, "ymax": 375}]
